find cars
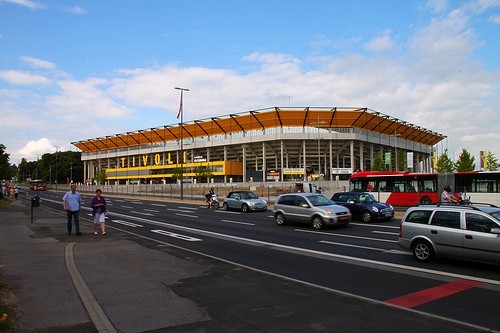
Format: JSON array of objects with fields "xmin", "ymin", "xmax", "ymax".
[
  {"xmin": 396, "ymin": 204, "xmax": 500, "ymax": 263},
  {"xmin": 273, "ymin": 193, "xmax": 351, "ymax": 229},
  {"xmin": 223, "ymin": 191, "xmax": 267, "ymax": 213},
  {"xmin": 330, "ymin": 192, "xmax": 394, "ymax": 222}
]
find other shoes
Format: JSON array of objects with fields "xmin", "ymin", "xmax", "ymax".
[
  {"xmin": 68, "ymin": 231, "xmax": 71, "ymax": 235},
  {"xmin": 103, "ymin": 232, "xmax": 106, "ymax": 235},
  {"xmin": 76, "ymin": 232, "xmax": 81, "ymax": 236},
  {"xmin": 94, "ymin": 232, "xmax": 98, "ymax": 234}
]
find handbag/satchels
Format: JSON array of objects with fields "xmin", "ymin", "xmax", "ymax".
[{"xmin": 104, "ymin": 209, "xmax": 108, "ymax": 215}]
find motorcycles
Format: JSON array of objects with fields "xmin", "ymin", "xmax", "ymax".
[{"xmin": 455, "ymin": 196, "xmax": 472, "ymax": 204}]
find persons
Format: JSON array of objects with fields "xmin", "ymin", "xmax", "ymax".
[
  {"xmin": 91, "ymin": 189, "xmax": 109, "ymax": 236},
  {"xmin": 0, "ymin": 179, "xmax": 19, "ymax": 201},
  {"xmin": 62, "ymin": 185, "xmax": 82, "ymax": 236},
  {"xmin": 206, "ymin": 187, "xmax": 214, "ymax": 209},
  {"xmin": 441, "ymin": 185, "xmax": 462, "ymax": 205}
]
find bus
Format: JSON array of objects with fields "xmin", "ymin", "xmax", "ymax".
[
  {"xmin": 348, "ymin": 171, "xmax": 500, "ymax": 209},
  {"xmin": 29, "ymin": 180, "xmax": 48, "ymax": 191}
]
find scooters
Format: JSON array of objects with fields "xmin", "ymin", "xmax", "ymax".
[{"xmin": 206, "ymin": 195, "xmax": 219, "ymax": 209}]
